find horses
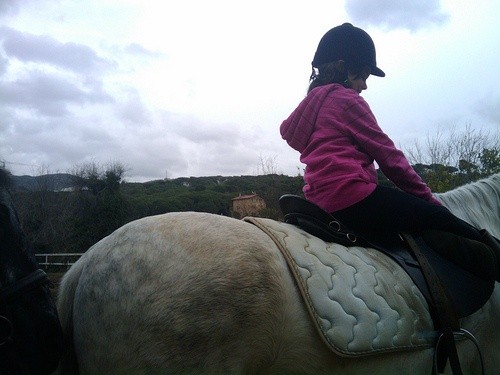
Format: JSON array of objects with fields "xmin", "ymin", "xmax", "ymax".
[
  {"xmin": 0, "ymin": 167, "xmax": 73, "ymax": 375},
  {"xmin": 55, "ymin": 171, "xmax": 500, "ymax": 374}
]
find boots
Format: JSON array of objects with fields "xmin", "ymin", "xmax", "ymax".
[{"xmin": 478, "ymin": 229, "xmax": 500, "ymax": 257}]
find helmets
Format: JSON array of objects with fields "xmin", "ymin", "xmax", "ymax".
[{"xmin": 313, "ymin": 23, "xmax": 385, "ymax": 77}]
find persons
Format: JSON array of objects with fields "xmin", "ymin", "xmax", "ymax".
[{"xmin": 281, "ymin": 23, "xmax": 500, "ymax": 285}]
